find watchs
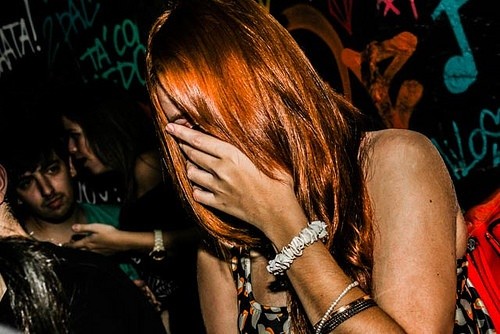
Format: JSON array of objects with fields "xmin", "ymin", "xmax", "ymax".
[{"xmin": 148, "ymin": 229, "xmax": 168, "ymax": 261}]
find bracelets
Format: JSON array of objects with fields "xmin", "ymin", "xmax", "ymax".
[
  {"xmin": 266, "ymin": 219, "xmax": 329, "ymax": 277},
  {"xmin": 313, "ymin": 280, "xmax": 379, "ymax": 334}
]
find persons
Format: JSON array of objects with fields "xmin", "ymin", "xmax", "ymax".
[
  {"xmin": 146, "ymin": 0, "xmax": 497, "ymax": 334},
  {"xmin": 0, "ymin": 77, "xmax": 205, "ymax": 334}
]
